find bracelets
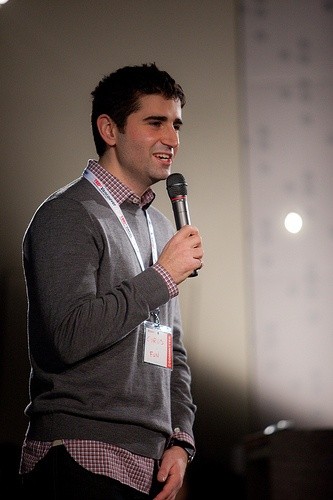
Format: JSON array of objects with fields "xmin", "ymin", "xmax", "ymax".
[{"xmin": 182, "ymin": 443, "xmax": 195, "ymax": 463}]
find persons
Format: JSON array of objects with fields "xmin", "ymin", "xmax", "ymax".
[{"xmin": 19, "ymin": 61, "xmax": 206, "ymax": 500}]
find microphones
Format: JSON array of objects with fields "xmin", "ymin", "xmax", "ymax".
[{"xmin": 165, "ymin": 173, "xmax": 198, "ymax": 278}]
